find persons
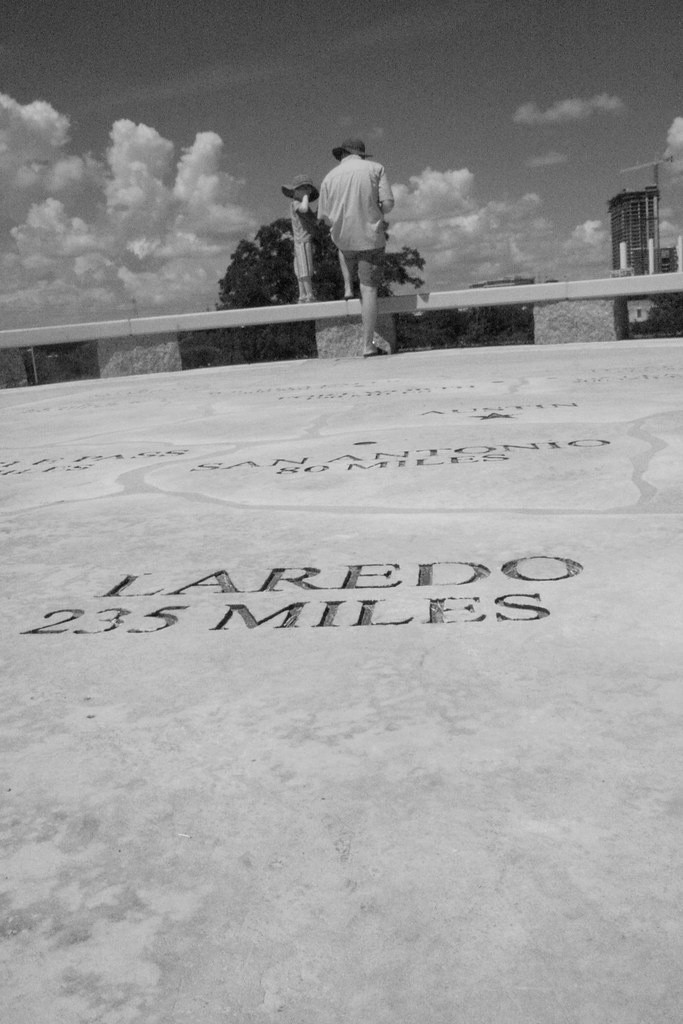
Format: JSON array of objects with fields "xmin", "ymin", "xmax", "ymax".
[
  {"xmin": 315, "ymin": 139, "xmax": 393, "ymax": 358},
  {"xmin": 281, "ymin": 174, "xmax": 319, "ymax": 302}
]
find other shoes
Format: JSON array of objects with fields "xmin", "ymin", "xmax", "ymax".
[{"xmin": 298, "ymin": 297, "xmax": 316, "ymax": 303}]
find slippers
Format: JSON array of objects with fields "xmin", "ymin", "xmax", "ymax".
[
  {"xmin": 345, "ymin": 293, "xmax": 355, "ymax": 300},
  {"xmin": 364, "ymin": 347, "xmax": 387, "ymax": 356}
]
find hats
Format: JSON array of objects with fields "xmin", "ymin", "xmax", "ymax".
[
  {"xmin": 332, "ymin": 138, "xmax": 373, "ymax": 161},
  {"xmin": 282, "ymin": 175, "xmax": 319, "ymax": 202}
]
